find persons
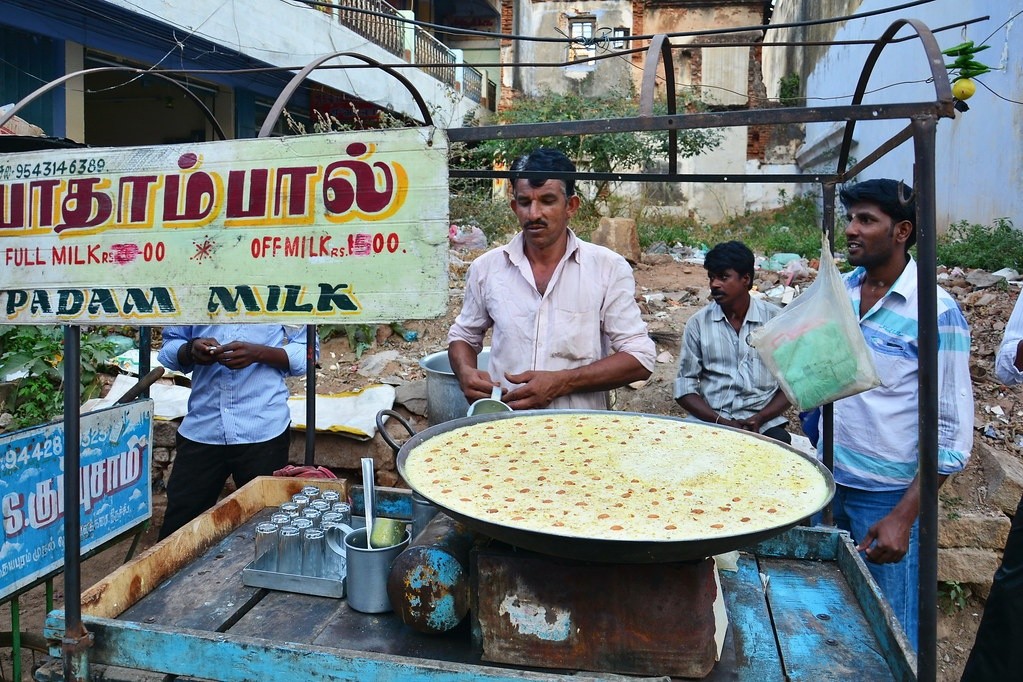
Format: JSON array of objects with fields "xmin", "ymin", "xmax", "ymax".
[
  {"xmin": 673, "ymin": 241, "xmax": 795, "ymax": 447},
  {"xmin": 449, "ymin": 148, "xmax": 657, "ymax": 411},
  {"xmin": 960, "ymin": 287, "xmax": 1023, "ymax": 682},
  {"xmin": 815, "ymin": 180, "xmax": 975, "ymax": 655},
  {"xmin": 157, "ymin": 323, "xmax": 321, "ymax": 545}
]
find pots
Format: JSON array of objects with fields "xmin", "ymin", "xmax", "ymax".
[{"xmin": 374, "ymin": 407, "xmax": 838, "ymax": 565}]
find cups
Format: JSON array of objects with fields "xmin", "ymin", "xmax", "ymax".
[
  {"xmin": 277, "ymin": 526, "xmax": 302, "ymax": 575},
  {"xmin": 326, "ymin": 523, "xmax": 411, "ymax": 613},
  {"xmin": 303, "ymin": 528, "xmax": 325, "ymax": 577},
  {"xmin": 318, "ymin": 521, "xmax": 342, "ymax": 579},
  {"xmin": 254, "ymin": 521, "xmax": 277, "ymax": 572},
  {"xmin": 370, "ymin": 518, "xmax": 407, "ymax": 548},
  {"xmin": 271, "ymin": 486, "xmax": 352, "ymax": 558}
]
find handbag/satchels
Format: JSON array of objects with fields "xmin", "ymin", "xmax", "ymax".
[{"xmin": 752, "ymin": 230, "xmax": 881, "ymax": 411}]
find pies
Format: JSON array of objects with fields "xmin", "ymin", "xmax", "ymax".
[{"xmin": 401, "ymin": 413, "xmax": 827, "ymax": 540}]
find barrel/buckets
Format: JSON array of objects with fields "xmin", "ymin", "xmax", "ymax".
[{"xmin": 419, "ymin": 346, "xmax": 494, "ymax": 427}]
